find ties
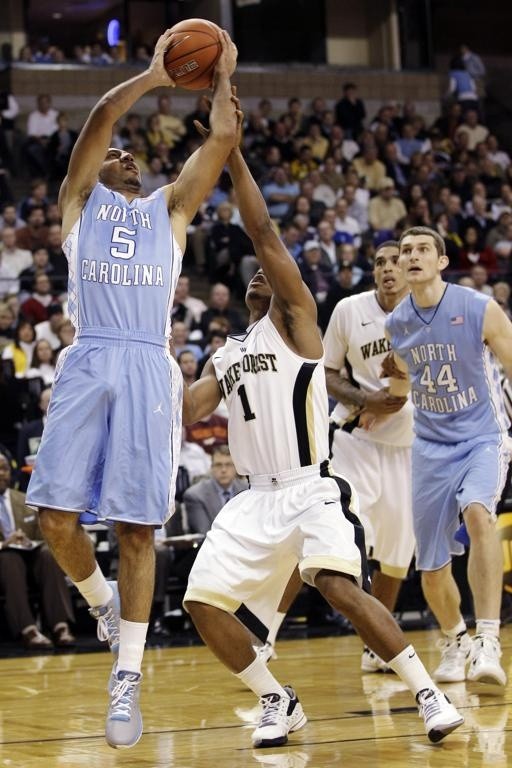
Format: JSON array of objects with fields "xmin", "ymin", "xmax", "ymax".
[
  {"xmin": 0, "ymin": 496, "xmax": 15, "ymax": 545},
  {"xmin": 222, "ymin": 490, "xmax": 233, "ymax": 506}
]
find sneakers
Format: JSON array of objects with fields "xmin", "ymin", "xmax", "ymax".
[
  {"xmin": 251, "ymin": 686, "xmax": 307, "ymax": 748},
  {"xmin": 89, "ymin": 579, "xmax": 124, "ymax": 659},
  {"xmin": 433, "ymin": 631, "xmax": 473, "ymax": 683},
  {"xmin": 468, "ymin": 634, "xmax": 506, "ymax": 695},
  {"xmin": 415, "ymin": 688, "xmax": 463, "ymax": 743},
  {"xmin": 103, "ymin": 670, "xmax": 144, "ymax": 749},
  {"xmin": 360, "ymin": 645, "xmax": 393, "ymax": 672}
]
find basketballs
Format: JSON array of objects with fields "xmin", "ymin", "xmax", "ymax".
[{"xmin": 164, "ymin": 18, "xmax": 222, "ymax": 89}]
